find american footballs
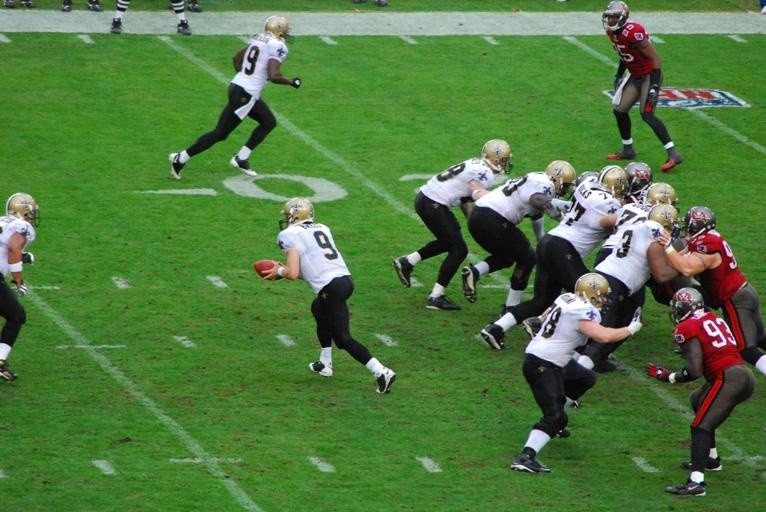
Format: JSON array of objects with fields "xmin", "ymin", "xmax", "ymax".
[{"xmin": 253, "ymin": 260, "xmax": 285, "ymax": 279}]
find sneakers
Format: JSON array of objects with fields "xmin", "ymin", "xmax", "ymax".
[
  {"xmin": 375, "ymin": 366, "xmax": 397, "ymax": 395},
  {"xmin": 3, "ymin": 0, "xmax": 205, "ymax": 36},
  {"xmin": 480, "ymin": 321, "xmax": 507, "ymax": 350},
  {"xmin": 392, "ymin": 254, "xmax": 415, "ymax": 288},
  {"xmin": 308, "ymin": 360, "xmax": 334, "ymax": 377},
  {"xmin": 425, "ymin": 294, "xmax": 462, "ymax": 312},
  {"xmin": 680, "ymin": 454, "xmax": 723, "ymax": 473},
  {"xmin": 0, "ymin": 359, "xmax": 18, "ymax": 382},
  {"xmin": 499, "ymin": 300, "xmax": 623, "ymax": 439},
  {"xmin": 664, "ymin": 475, "xmax": 708, "ymax": 497},
  {"xmin": 510, "ymin": 452, "xmax": 552, "ymax": 474},
  {"xmin": 168, "ymin": 152, "xmax": 186, "ymax": 181},
  {"xmin": 461, "ymin": 263, "xmax": 481, "ymax": 303},
  {"xmin": 230, "ymin": 154, "xmax": 258, "ymax": 177},
  {"xmin": 661, "ymin": 154, "xmax": 685, "ymax": 172},
  {"xmin": 607, "ymin": 148, "xmax": 636, "ymax": 161}
]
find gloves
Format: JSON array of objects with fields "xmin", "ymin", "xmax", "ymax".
[
  {"xmin": 292, "ymin": 78, "xmax": 302, "ymax": 88},
  {"xmin": 646, "ymin": 362, "xmax": 671, "ymax": 384},
  {"xmin": 613, "ymin": 75, "xmax": 624, "ymax": 92},
  {"xmin": 648, "ymin": 84, "xmax": 660, "ymax": 107},
  {"xmin": 11, "ymin": 279, "xmax": 28, "ymax": 297},
  {"xmin": 22, "ymin": 251, "xmax": 34, "ymax": 265},
  {"xmin": 626, "ymin": 315, "xmax": 643, "ymax": 336}
]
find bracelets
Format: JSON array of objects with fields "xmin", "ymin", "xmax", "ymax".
[
  {"xmin": 276, "ymin": 267, "xmax": 285, "ymax": 278},
  {"xmin": 9, "ymin": 261, "xmax": 23, "ymax": 275}
]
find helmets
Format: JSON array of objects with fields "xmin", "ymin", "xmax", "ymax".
[
  {"xmin": 5, "ymin": 191, "xmax": 41, "ymax": 229},
  {"xmin": 576, "ymin": 161, "xmax": 680, "ymax": 244},
  {"xmin": 574, "ymin": 272, "xmax": 612, "ymax": 315},
  {"xmin": 668, "ymin": 287, "xmax": 706, "ymax": 328},
  {"xmin": 481, "ymin": 139, "xmax": 514, "ymax": 175},
  {"xmin": 279, "ymin": 196, "xmax": 315, "ymax": 232},
  {"xmin": 544, "ymin": 160, "xmax": 577, "ymax": 201},
  {"xmin": 264, "ymin": 14, "xmax": 296, "ymax": 45},
  {"xmin": 680, "ymin": 206, "xmax": 717, "ymax": 242},
  {"xmin": 601, "ymin": 0, "xmax": 630, "ymax": 32}
]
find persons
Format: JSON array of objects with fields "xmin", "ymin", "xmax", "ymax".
[
  {"xmin": 0, "ymin": 192, "xmax": 37, "ymax": 380},
  {"xmin": 601, "ymin": 2, "xmax": 685, "ymax": 171},
  {"xmin": 261, "ymin": 197, "xmax": 396, "ymax": 394},
  {"xmin": 168, "ymin": 17, "xmax": 303, "ymax": 178},
  {"xmin": 0, "ymin": 0, "xmax": 202, "ymax": 37}
]
